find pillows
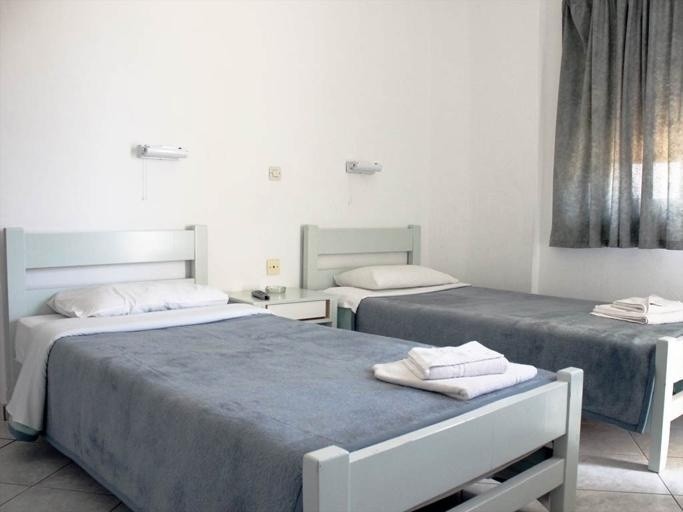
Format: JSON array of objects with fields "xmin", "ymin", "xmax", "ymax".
[
  {"xmin": 334, "ymin": 265, "xmax": 459, "ymax": 290},
  {"xmin": 46, "ymin": 279, "xmax": 228, "ymax": 318}
]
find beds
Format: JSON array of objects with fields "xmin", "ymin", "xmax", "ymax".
[
  {"xmin": 300, "ymin": 224, "xmax": 683, "ymax": 474},
  {"xmin": 6, "ymin": 225, "xmax": 583, "ymax": 509}
]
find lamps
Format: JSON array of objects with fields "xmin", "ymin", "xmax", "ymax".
[
  {"xmin": 345, "ymin": 160, "xmax": 384, "ymax": 175},
  {"xmin": 136, "ymin": 143, "xmax": 190, "ymax": 162}
]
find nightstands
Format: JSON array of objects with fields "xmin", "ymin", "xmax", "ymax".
[{"xmin": 227, "ymin": 287, "xmax": 336, "ymax": 333}]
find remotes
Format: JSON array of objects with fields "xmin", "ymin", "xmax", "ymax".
[{"xmin": 251, "ymin": 290, "xmax": 270, "ymax": 300}]
